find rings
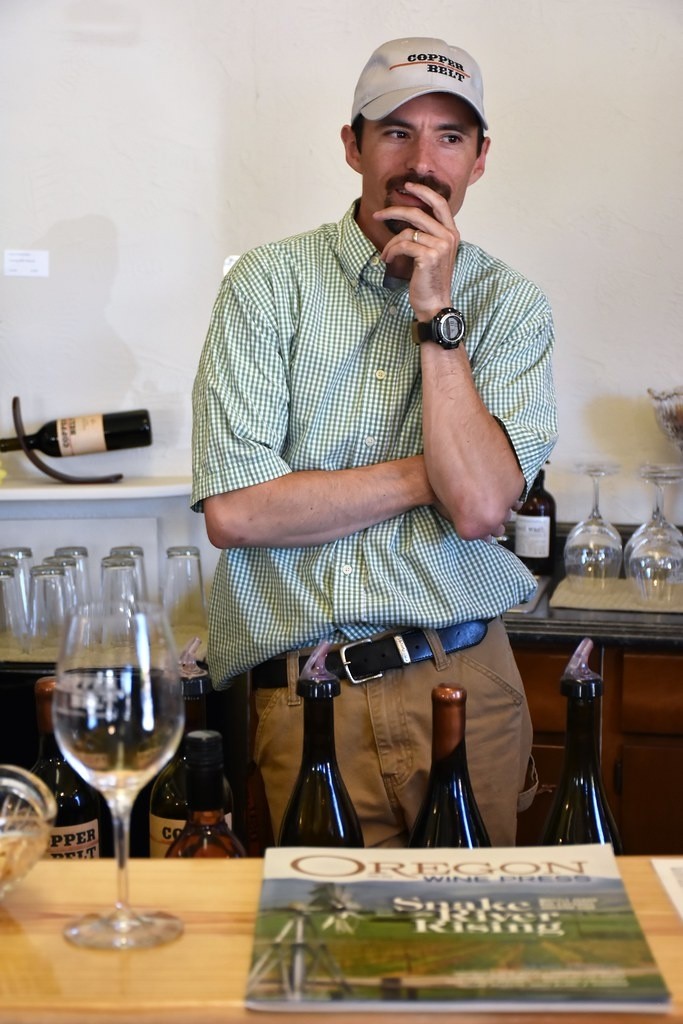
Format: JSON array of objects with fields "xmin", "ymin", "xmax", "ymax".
[{"xmin": 413, "ymin": 230, "xmax": 419, "ymax": 242}]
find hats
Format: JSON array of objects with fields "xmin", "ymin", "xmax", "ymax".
[{"xmin": 350, "ymin": 37, "xmax": 489, "ymax": 138}]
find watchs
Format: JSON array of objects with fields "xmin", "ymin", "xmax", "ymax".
[{"xmin": 411, "ymin": 307, "xmax": 466, "ymax": 350}]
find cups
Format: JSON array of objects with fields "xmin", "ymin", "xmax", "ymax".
[{"xmin": 0, "ymin": 546, "xmax": 209, "ymax": 657}]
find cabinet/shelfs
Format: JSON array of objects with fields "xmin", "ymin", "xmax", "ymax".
[
  {"xmin": 510, "ymin": 643, "xmax": 683, "ymax": 857},
  {"xmin": 0, "ymin": 480, "xmax": 217, "ymax": 702}
]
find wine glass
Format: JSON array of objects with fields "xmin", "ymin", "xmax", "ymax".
[
  {"xmin": 51, "ymin": 602, "xmax": 184, "ymax": 952},
  {"xmin": 561, "ymin": 458, "xmax": 683, "ymax": 602}
]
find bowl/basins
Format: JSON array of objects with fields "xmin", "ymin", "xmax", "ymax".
[
  {"xmin": 0, "ymin": 766, "xmax": 57, "ymax": 906},
  {"xmin": 646, "ymin": 385, "xmax": 683, "ymax": 456}
]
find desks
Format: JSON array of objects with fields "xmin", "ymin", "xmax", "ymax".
[{"xmin": 0, "ymin": 855, "xmax": 682, "ymax": 1024}]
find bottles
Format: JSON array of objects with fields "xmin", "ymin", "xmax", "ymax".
[
  {"xmin": 514, "ymin": 470, "xmax": 556, "ymax": 579},
  {"xmin": 33, "ymin": 668, "xmax": 245, "ymax": 859},
  {"xmin": 0, "ymin": 410, "xmax": 153, "ymax": 457},
  {"xmin": 408, "ymin": 682, "xmax": 492, "ymax": 848},
  {"xmin": 543, "ymin": 676, "xmax": 624, "ymax": 856},
  {"xmin": 277, "ymin": 671, "xmax": 364, "ymax": 848}
]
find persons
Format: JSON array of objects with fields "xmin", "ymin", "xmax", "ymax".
[{"xmin": 190, "ymin": 37, "xmax": 560, "ymax": 849}]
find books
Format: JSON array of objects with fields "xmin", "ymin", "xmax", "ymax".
[{"xmin": 246, "ymin": 840, "xmax": 671, "ymax": 1013}]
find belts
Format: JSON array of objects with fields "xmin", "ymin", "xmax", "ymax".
[{"xmin": 249, "ymin": 615, "xmax": 496, "ymax": 689}]
